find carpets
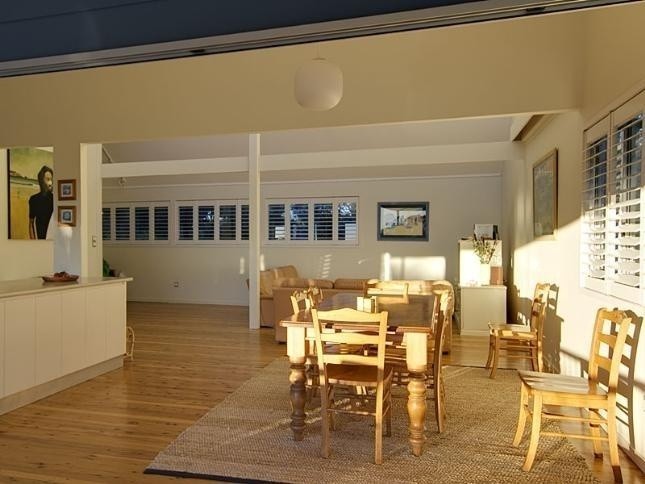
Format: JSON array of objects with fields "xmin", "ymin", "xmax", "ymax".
[{"xmin": 143, "ymin": 351, "xmax": 599, "ymax": 484}]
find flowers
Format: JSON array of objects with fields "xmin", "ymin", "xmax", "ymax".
[{"xmin": 471, "ymin": 233, "xmax": 498, "ymax": 264}]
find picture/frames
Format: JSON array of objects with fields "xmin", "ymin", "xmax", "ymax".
[
  {"xmin": 6, "ymin": 147, "xmax": 54, "ymax": 241},
  {"xmin": 57, "ymin": 206, "xmax": 76, "ymax": 227},
  {"xmin": 531, "ymin": 147, "xmax": 559, "ymax": 235},
  {"xmin": 57, "ymin": 180, "xmax": 76, "ymax": 200},
  {"xmin": 377, "ymin": 201, "xmax": 429, "ymax": 240}
]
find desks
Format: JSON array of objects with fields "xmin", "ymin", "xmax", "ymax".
[{"xmin": 278, "ymin": 293, "xmax": 435, "ymax": 443}]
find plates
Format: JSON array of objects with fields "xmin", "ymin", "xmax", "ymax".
[{"xmin": 42, "ymin": 275, "xmax": 79, "ymax": 282}]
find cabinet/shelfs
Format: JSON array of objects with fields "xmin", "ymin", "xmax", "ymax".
[
  {"xmin": 456, "ymin": 239, "xmax": 508, "ymax": 335},
  {"xmin": 63, "ymin": 282, "xmax": 127, "ymax": 388},
  {"xmin": 1, "ymin": 292, "xmax": 62, "ymax": 417}
]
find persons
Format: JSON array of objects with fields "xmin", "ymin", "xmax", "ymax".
[{"xmin": 27, "ymin": 165, "xmax": 55, "ymax": 240}]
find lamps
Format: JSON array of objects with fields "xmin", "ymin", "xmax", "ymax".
[{"xmin": 293, "ymin": 42, "xmax": 344, "ymax": 110}]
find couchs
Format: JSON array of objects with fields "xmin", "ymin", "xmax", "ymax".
[{"xmin": 246, "ymin": 264, "xmax": 455, "ymax": 352}]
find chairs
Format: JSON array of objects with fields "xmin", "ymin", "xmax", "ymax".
[
  {"xmin": 392, "ymin": 294, "xmax": 449, "ymax": 386},
  {"xmin": 485, "ymin": 295, "xmax": 546, "ymax": 377},
  {"xmin": 288, "ymin": 291, "xmax": 353, "ymax": 403},
  {"xmin": 386, "ymin": 300, "xmax": 449, "ymax": 432},
  {"xmin": 512, "ymin": 306, "xmax": 631, "ymax": 484},
  {"xmin": 307, "ymin": 287, "xmax": 362, "ymax": 397},
  {"xmin": 311, "ymin": 308, "xmax": 394, "ymax": 464}
]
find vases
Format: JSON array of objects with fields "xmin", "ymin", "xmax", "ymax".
[{"xmin": 479, "ymin": 264, "xmax": 490, "ymax": 286}]
